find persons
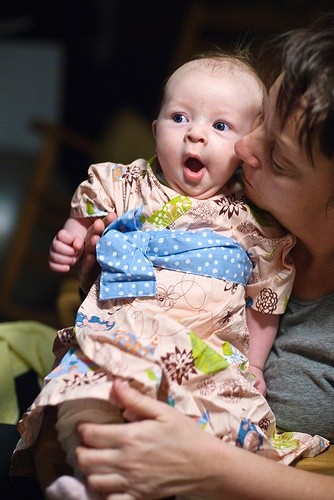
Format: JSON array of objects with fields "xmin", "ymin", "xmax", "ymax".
[
  {"xmin": 46, "ymin": 44, "xmax": 296, "ymax": 500},
  {"xmin": 53, "ymin": 28, "xmax": 334, "ymax": 499}
]
